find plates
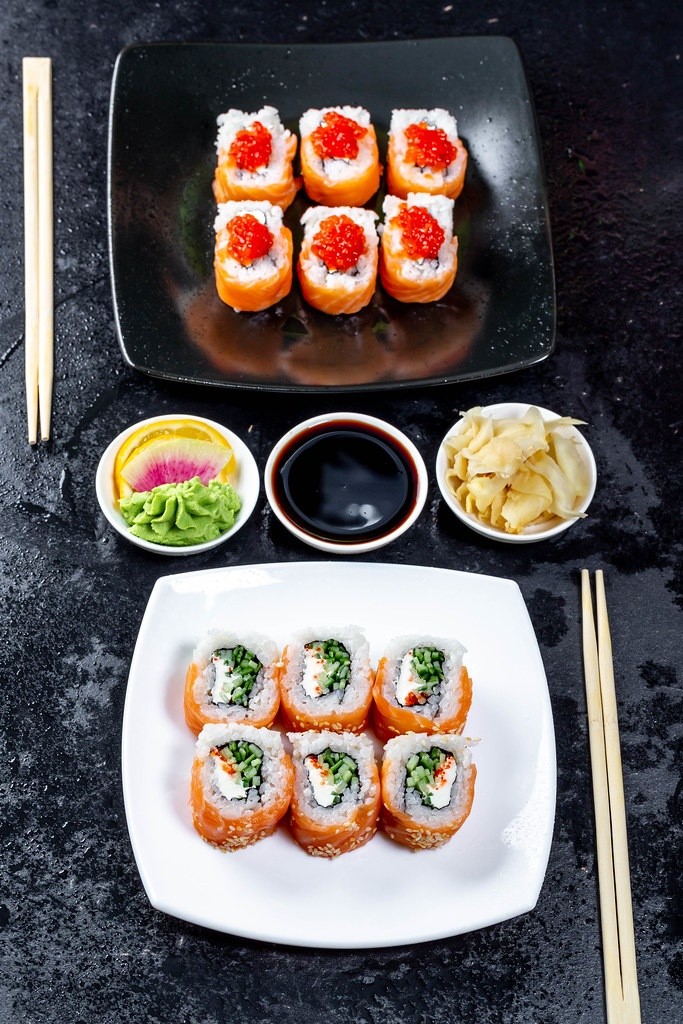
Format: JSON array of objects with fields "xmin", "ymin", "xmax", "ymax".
[
  {"xmin": 107, "ymin": 38, "xmax": 559, "ymax": 396},
  {"xmin": 95, "ymin": 412, "xmax": 260, "ymax": 554},
  {"xmin": 436, "ymin": 400, "xmax": 596, "ymax": 544},
  {"xmin": 265, "ymin": 410, "xmax": 429, "ymax": 553},
  {"xmin": 121, "ymin": 560, "xmax": 554, "ymax": 952}
]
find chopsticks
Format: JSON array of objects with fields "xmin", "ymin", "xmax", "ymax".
[
  {"xmin": 21, "ymin": 57, "xmax": 54, "ymax": 443},
  {"xmin": 581, "ymin": 567, "xmax": 643, "ymax": 1024}
]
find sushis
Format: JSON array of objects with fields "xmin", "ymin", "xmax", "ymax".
[
  {"xmin": 184, "ymin": 624, "xmax": 476, "ymax": 858},
  {"xmin": 214, "ymin": 103, "xmax": 468, "ymax": 311}
]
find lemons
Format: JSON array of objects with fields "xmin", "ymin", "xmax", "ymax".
[{"xmin": 114, "ymin": 418, "xmax": 236, "ymax": 498}]
